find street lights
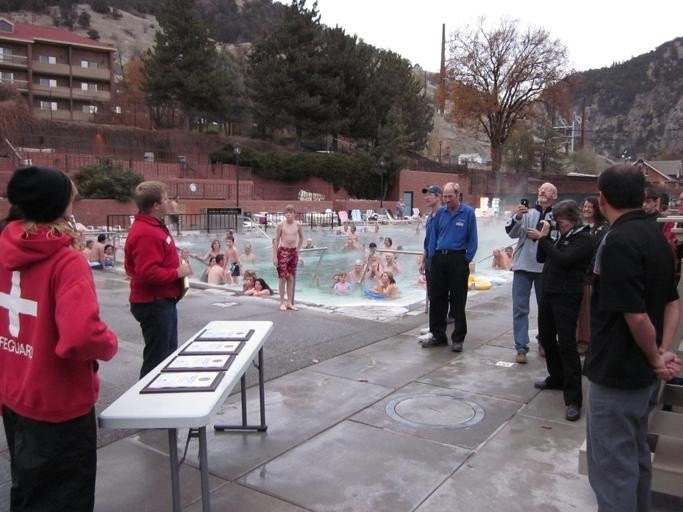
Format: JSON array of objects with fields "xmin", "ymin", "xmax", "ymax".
[
  {"xmin": 233, "ymin": 142, "xmax": 240, "ymax": 208},
  {"xmin": 621, "ymin": 150, "xmax": 631, "ymax": 166},
  {"xmin": 378, "ymin": 160, "xmax": 386, "ymax": 208},
  {"xmin": 438, "ymin": 140, "xmax": 442, "ymax": 164}
]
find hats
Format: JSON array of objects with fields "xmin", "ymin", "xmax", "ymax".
[{"xmin": 7, "ymin": 166, "xmax": 72, "ymax": 222}]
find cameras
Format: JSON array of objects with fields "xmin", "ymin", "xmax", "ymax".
[{"xmin": 536, "ymin": 219, "xmax": 558, "ymax": 232}]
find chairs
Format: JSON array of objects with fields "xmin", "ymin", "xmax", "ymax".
[{"xmin": 243, "ymin": 207, "xmax": 421, "ymax": 227}]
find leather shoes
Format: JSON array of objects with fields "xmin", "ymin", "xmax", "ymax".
[
  {"xmin": 566, "ymin": 405, "xmax": 581, "ymax": 421},
  {"xmin": 452, "ymin": 341, "xmax": 463, "ymax": 352},
  {"xmin": 421, "ymin": 336, "xmax": 449, "ymax": 347},
  {"xmin": 534, "ymin": 380, "xmax": 560, "ymax": 390}
]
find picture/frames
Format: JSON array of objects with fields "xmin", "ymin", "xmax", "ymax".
[
  {"xmin": 162, "ymin": 356, "xmax": 236, "ymax": 371},
  {"xmin": 139, "ymin": 372, "xmax": 224, "ymax": 392},
  {"xmin": 194, "ymin": 326, "xmax": 254, "ymax": 341},
  {"xmin": 179, "ymin": 342, "xmax": 247, "ymax": 355}
]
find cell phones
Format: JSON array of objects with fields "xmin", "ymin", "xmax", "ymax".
[{"xmin": 521, "ymin": 198, "xmax": 529, "ymax": 213}]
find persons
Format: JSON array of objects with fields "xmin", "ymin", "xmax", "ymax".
[
  {"xmin": 503, "ymin": 181, "xmax": 559, "ymax": 365},
  {"xmin": 504, "ymin": 246, "xmax": 514, "ymax": 257},
  {"xmin": 0, "ymin": 164, "xmax": 119, "ymax": 512},
  {"xmin": 396, "ymin": 198, "xmax": 405, "ymax": 219},
  {"xmin": 577, "ymin": 185, "xmax": 683, "ymax": 356},
  {"xmin": 273, "ymin": 205, "xmax": 303, "ymax": 312},
  {"xmin": 82, "ymin": 240, "xmax": 94, "ymax": 263},
  {"xmin": 396, "ymin": 202, "xmax": 400, "ymax": 219},
  {"xmin": 124, "ymin": 180, "xmax": 191, "ymax": 381},
  {"xmin": 103, "ymin": 243, "xmax": 117, "ymax": 267},
  {"xmin": 419, "ymin": 182, "xmax": 478, "ymax": 351},
  {"xmin": 92, "ymin": 233, "xmax": 105, "ymax": 269},
  {"xmin": 584, "ymin": 165, "xmax": 683, "ymax": 512},
  {"xmin": 176, "ymin": 214, "xmax": 427, "ymax": 297},
  {"xmin": 526, "ymin": 198, "xmax": 595, "ymax": 421},
  {"xmin": 421, "ymin": 185, "xmax": 455, "ymax": 325},
  {"xmin": 492, "ymin": 248, "xmax": 512, "ymax": 270},
  {"xmin": 169, "ymin": 195, "xmax": 183, "ymax": 235},
  {"xmin": 467, "ymin": 261, "xmax": 477, "ymax": 291}
]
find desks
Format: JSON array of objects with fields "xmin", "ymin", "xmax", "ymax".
[{"xmin": 98, "ymin": 321, "xmax": 275, "ymax": 512}]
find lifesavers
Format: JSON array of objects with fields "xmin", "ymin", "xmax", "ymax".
[
  {"xmin": 362, "ymin": 281, "xmax": 385, "ymax": 299},
  {"xmin": 468, "ymin": 281, "xmax": 491, "ymax": 289},
  {"xmin": 332, "ymin": 282, "xmax": 353, "ymax": 296}
]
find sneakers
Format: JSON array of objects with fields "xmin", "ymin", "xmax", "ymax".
[
  {"xmin": 418, "ymin": 333, "xmax": 433, "ymax": 342},
  {"xmin": 516, "ymin": 352, "xmax": 528, "ymax": 363},
  {"xmin": 420, "ymin": 327, "xmax": 431, "ymax": 333}
]
